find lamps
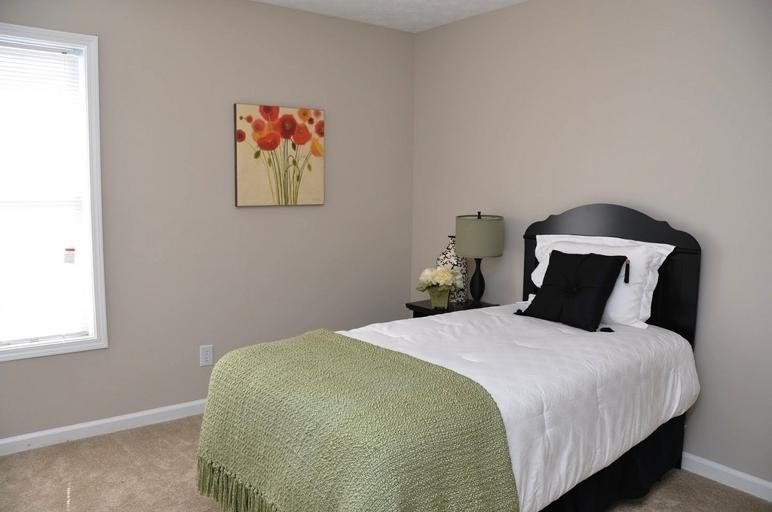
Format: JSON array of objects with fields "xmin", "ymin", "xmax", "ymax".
[{"xmin": 455, "ymin": 212, "xmax": 504, "ymax": 309}]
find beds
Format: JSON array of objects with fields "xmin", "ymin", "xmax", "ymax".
[{"xmin": 199, "ymin": 202, "xmax": 701, "ymax": 512}]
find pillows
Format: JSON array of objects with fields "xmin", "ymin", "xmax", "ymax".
[
  {"xmin": 530, "ymin": 233, "xmax": 678, "ymax": 330},
  {"xmin": 513, "ymin": 250, "xmax": 630, "ymax": 332}
]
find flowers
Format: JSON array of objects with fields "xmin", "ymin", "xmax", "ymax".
[{"xmin": 415, "ymin": 267, "xmax": 466, "ymax": 294}]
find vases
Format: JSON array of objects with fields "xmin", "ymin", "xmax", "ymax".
[
  {"xmin": 437, "ymin": 235, "xmax": 469, "ymax": 305},
  {"xmin": 429, "ymin": 285, "xmax": 453, "ymax": 311}
]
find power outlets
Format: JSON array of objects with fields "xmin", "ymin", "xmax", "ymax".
[{"xmin": 200, "ymin": 344, "xmax": 215, "ymax": 368}]
